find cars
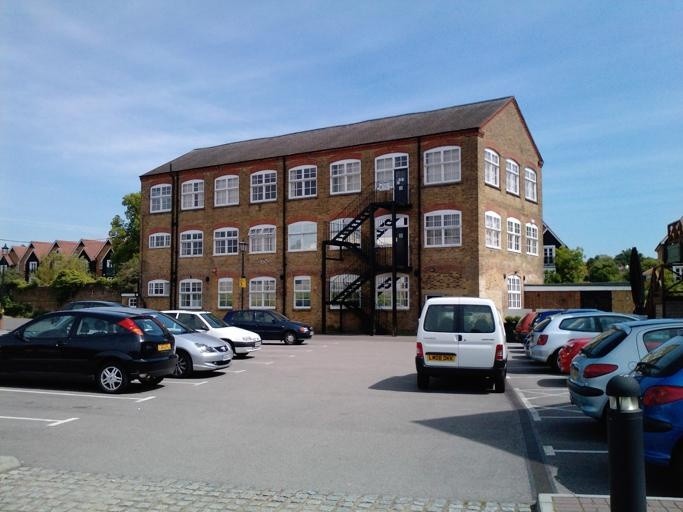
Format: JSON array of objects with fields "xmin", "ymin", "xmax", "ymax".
[
  {"xmin": 221, "ymin": 308, "xmax": 314, "ymax": 345},
  {"xmin": 0, "ymin": 308, "xmax": 180, "ymax": 394},
  {"xmin": 52, "ymin": 307, "xmax": 234, "ymax": 379},
  {"xmin": 513, "ymin": 308, "xmax": 683, "ymax": 474},
  {"xmin": 143, "ymin": 309, "xmax": 263, "ymax": 358},
  {"xmin": 51, "ymin": 299, "xmax": 129, "ymax": 324}
]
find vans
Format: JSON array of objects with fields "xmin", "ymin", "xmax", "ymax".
[{"xmin": 415, "ymin": 296, "xmax": 509, "ymax": 393}]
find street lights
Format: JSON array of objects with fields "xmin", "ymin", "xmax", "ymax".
[{"xmin": 239, "ymin": 237, "xmax": 248, "ymax": 309}]
